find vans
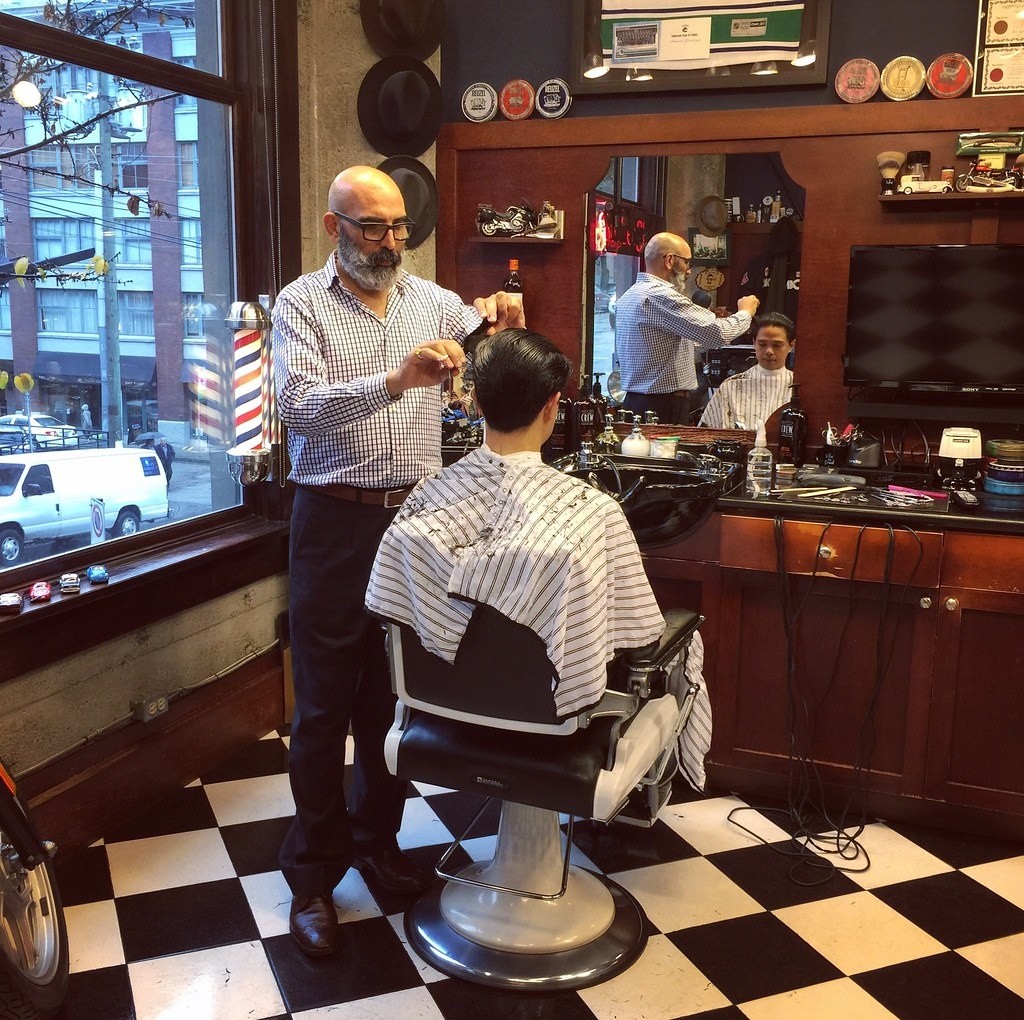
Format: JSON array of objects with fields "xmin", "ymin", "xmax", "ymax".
[{"xmin": 0, "ymin": 446, "xmax": 171, "ymax": 564}]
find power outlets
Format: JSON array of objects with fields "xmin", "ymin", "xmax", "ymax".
[{"xmin": 129, "ymin": 690, "xmax": 169, "ymax": 723}]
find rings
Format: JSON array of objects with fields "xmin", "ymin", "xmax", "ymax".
[{"xmin": 414, "ymin": 348, "xmax": 424, "ymax": 360}]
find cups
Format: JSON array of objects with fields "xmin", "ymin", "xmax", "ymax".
[{"xmin": 815, "ymin": 444, "xmax": 847, "ymax": 470}]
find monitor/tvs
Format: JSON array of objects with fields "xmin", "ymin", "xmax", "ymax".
[{"xmin": 843, "ymin": 243, "xmax": 1024, "ymax": 395}]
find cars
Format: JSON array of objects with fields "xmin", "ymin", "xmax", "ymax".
[
  {"xmin": 1, "ymin": 413, "xmax": 77, "ymax": 448},
  {"xmin": 125, "ymin": 399, "xmax": 158, "ymax": 429},
  {"xmin": 595, "ymin": 284, "xmax": 611, "ymax": 311},
  {"xmin": 607, "ymin": 284, "xmax": 617, "ymax": 330}
]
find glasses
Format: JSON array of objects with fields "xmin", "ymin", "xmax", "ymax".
[
  {"xmin": 334, "ymin": 211, "xmax": 415, "ymax": 242},
  {"xmin": 663, "ymin": 253, "xmax": 694, "ymax": 269}
]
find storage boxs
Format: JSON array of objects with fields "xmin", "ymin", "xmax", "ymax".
[{"xmin": 283, "ymin": 646, "xmax": 296, "ymax": 723}]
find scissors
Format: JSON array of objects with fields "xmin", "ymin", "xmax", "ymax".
[
  {"xmin": 850, "ymin": 493, "xmax": 869, "ymax": 503},
  {"xmin": 437, "ymin": 354, "xmax": 473, "ymax": 370},
  {"xmin": 843, "ymin": 423, "xmax": 864, "ymax": 442},
  {"xmin": 821, "ymin": 427, "xmax": 837, "ymax": 440},
  {"xmin": 814, "ymin": 491, "xmax": 850, "ymax": 504},
  {"xmin": 869, "ymin": 487, "xmax": 935, "ymax": 507}
]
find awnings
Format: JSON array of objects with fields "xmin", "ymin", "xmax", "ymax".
[{"xmin": 32, "ymin": 352, "xmax": 157, "ymax": 392}]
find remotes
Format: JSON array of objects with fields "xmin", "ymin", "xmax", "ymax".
[{"xmin": 953, "ymin": 491, "xmax": 980, "ymax": 507}]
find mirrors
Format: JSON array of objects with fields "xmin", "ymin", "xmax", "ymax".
[{"xmin": 580, "ymin": 151, "xmax": 806, "ymax": 446}]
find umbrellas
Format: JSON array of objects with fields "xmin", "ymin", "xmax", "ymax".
[{"xmin": 136, "ymin": 429, "xmax": 163, "ymax": 440}]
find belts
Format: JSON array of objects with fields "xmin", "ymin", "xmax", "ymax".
[
  {"xmin": 628, "ymin": 391, "xmax": 693, "ymax": 400},
  {"xmin": 298, "ymin": 483, "xmax": 414, "ymax": 509}
]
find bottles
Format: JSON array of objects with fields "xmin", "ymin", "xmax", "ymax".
[
  {"xmin": 775, "ymin": 191, "xmax": 781, "ymax": 201},
  {"xmin": 504, "ymin": 259, "xmax": 523, "ymax": 304},
  {"xmin": 745, "ymin": 203, "xmax": 762, "ymax": 223}
]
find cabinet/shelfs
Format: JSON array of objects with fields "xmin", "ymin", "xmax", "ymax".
[{"xmin": 636, "ymin": 501, "xmax": 1024, "ymax": 828}]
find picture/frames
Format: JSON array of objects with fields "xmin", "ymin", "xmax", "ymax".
[
  {"xmin": 687, "ymin": 226, "xmax": 729, "ymax": 266},
  {"xmin": 440, "ymin": 349, "xmax": 486, "ymax": 450},
  {"xmin": 569, "ymin": 0, "xmax": 831, "ymax": 96}
]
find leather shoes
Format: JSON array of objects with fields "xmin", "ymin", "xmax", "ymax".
[
  {"xmin": 349, "ymin": 836, "xmax": 428, "ymax": 895},
  {"xmin": 290, "ymin": 891, "xmax": 342, "ymax": 957}
]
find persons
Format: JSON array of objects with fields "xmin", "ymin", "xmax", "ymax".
[
  {"xmin": 697, "ymin": 312, "xmax": 794, "ymax": 431},
  {"xmin": 616, "ymin": 232, "xmax": 761, "ymax": 427},
  {"xmin": 268, "ymin": 166, "xmax": 526, "ymax": 954},
  {"xmin": 82, "ymin": 404, "xmax": 92, "ymax": 443},
  {"xmin": 401, "ymin": 329, "xmax": 703, "ymax": 813},
  {"xmin": 141, "ymin": 439, "xmax": 157, "ymax": 453},
  {"xmin": 155, "ymin": 438, "xmax": 175, "ymax": 490}
]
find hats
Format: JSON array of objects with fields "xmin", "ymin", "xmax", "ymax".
[
  {"xmin": 377, "ymin": 154, "xmax": 439, "ymax": 250},
  {"xmin": 82, "ymin": 404, "xmax": 88, "ymax": 411},
  {"xmin": 360, "ymin": 0, "xmax": 445, "ymax": 61},
  {"xmin": 356, "ymin": 54, "xmax": 445, "ymax": 158},
  {"xmin": 696, "ymin": 196, "xmax": 729, "ymax": 238},
  {"xmin": 769, "ymin": 217, "xmax": 799, "ymax": 257}
]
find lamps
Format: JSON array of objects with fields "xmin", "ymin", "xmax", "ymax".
[{"xmin": 583, "ymin": 20, "xmax": 817, "ymax": 83}]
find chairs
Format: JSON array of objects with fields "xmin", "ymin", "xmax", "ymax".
[{"xmin": 382, "ymin": 524, "xmax": 709, "ymax": 991}]
